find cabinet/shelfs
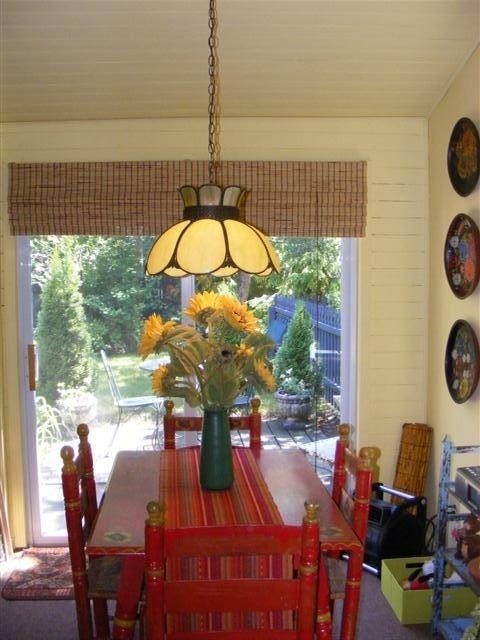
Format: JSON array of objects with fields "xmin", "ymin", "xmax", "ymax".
[{"xmin": 426, "ymin": 435, "xmax": 480, "ymax": 639}]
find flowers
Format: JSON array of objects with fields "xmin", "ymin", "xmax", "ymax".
[{"xmin": 139, "ymin": 288, "xmax": 279, "ymax": 411}]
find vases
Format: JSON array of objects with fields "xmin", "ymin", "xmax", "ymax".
[{"xmin": 201, "ymin": 405, "xmax": 233, "ymax": 492}]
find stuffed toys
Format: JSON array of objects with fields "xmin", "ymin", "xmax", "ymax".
[{"xmin": 400, "ymin": 516, "xmax": 480, "ymax": 590}]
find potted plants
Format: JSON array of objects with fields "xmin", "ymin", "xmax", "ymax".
[{"xmin": 272, "ymin": 307, "xmax": 324, "ymax": 427}]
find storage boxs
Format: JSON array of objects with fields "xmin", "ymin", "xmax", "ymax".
[{"xmin": 381, "ymin": 555, "xmax": 479, "ymax": 627}]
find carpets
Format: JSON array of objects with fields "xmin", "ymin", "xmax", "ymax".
[{"xmin": 2, "ymin": 544, "xmax": 84, "ymax": 599}]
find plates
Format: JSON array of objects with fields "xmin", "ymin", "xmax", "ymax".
[
  {"xmin": 443, "ymin": 318, "xmax": 480, "ymax": 405},
  {"xmin": 443, "ymin": 211, "xmax": 480, "ymax": 302},
  {"xmin": 445, "ymin": 116, "xmax": 478, "ymax": 199}
]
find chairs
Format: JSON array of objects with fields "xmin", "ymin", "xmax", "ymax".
[
  {"xmin": 143, "ymin": 502, "xmax": 321, "ymax": 640},
  {"xmin": 228, "ymin": 352, "xmax": 283, "ymax": 448},
  {"xmin": 161, "ymin": 398, "xmax": 262, "ymax": 447},
  {"xmin": 321, "ymin": 422, "xmax": 376, "ymax": 640},
  {"xmin": 59, "ymin": 421, "xmax": 145, "ymax": 638},
  {"xmin": 100, "ymin": 348, "xmax": 164, "ymax": 457}
]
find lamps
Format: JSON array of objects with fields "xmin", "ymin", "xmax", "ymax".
[{"xmin": 139, "ymin": 1, "xmax": 287, "ymax": 278}]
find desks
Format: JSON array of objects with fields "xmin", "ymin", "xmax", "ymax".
[
  {"xmin": 137, "ymin": 355, "xmax": 223, "ymax": 448},
  {"xmin": 87, "ymin": 448, "xmax": 363, "ymax": 640}
]
